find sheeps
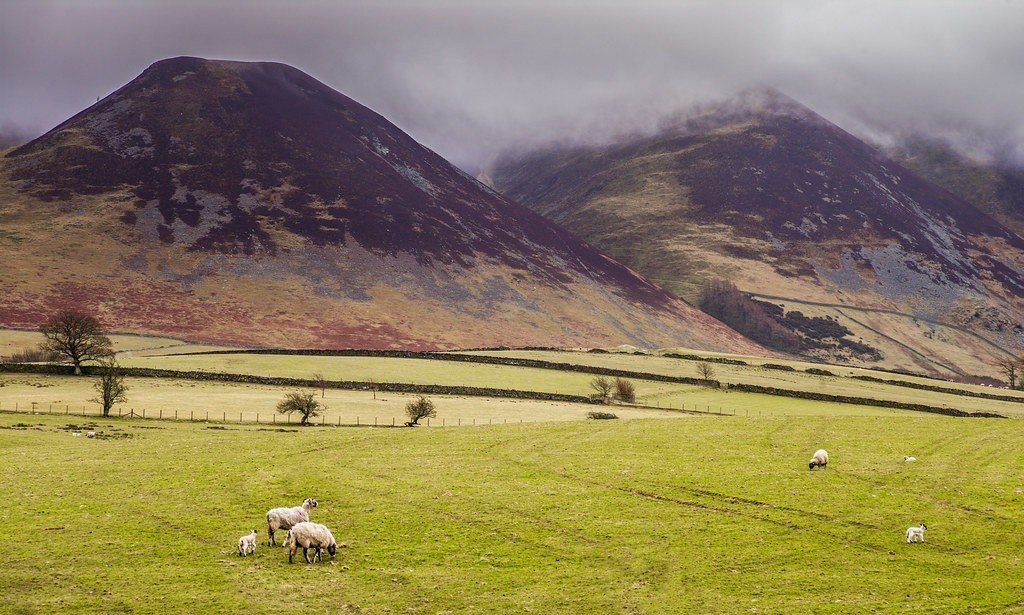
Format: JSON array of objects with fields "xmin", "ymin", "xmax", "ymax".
[
  {"xmin": 905, "ymin": 522, "xmax": 929, "ymax": 544},
  {"xmin": 237, "ymin": 530, "xmax": 259, "ymax": 558},
  {"xmin": 281, "ymin": 521, "xmax": 337, "ymax": 564},
  {"xmin": 903, "ymin": 455, "xmax": 916, "ymax": 461},
  {"xmin": 266, "ymin": 498, "xmax": 318, "ymax": 547},
  {"xmin": 809, "ymin": 449, "xmax": 831, "ymax": 470}
]
